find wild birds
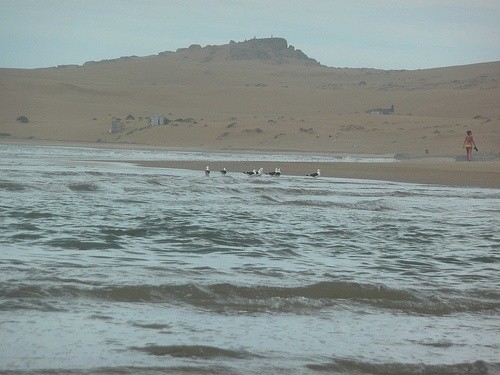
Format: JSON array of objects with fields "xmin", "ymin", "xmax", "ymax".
[
  {"xmin": 219, "ymin": 167, "xmax": 227, "ymax": 176},
  {"xmin": 264, "ymin": 167, "xmax": 281, "ymax": 177},
  {"xmin": 305, "ymin": 167, "xmax": 321, "ymax": 180},
  {"xmin": 242, "ymin": 167, "xmax": 264, "ymax": 178},
  {"xmin": 203, "ymin": 165, "xmax": 210, "ymax": 177}
]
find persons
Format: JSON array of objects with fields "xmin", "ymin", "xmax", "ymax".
[{"xmin": 463, "ymin": 131, "xmax": 476, "ymax": 161}]
